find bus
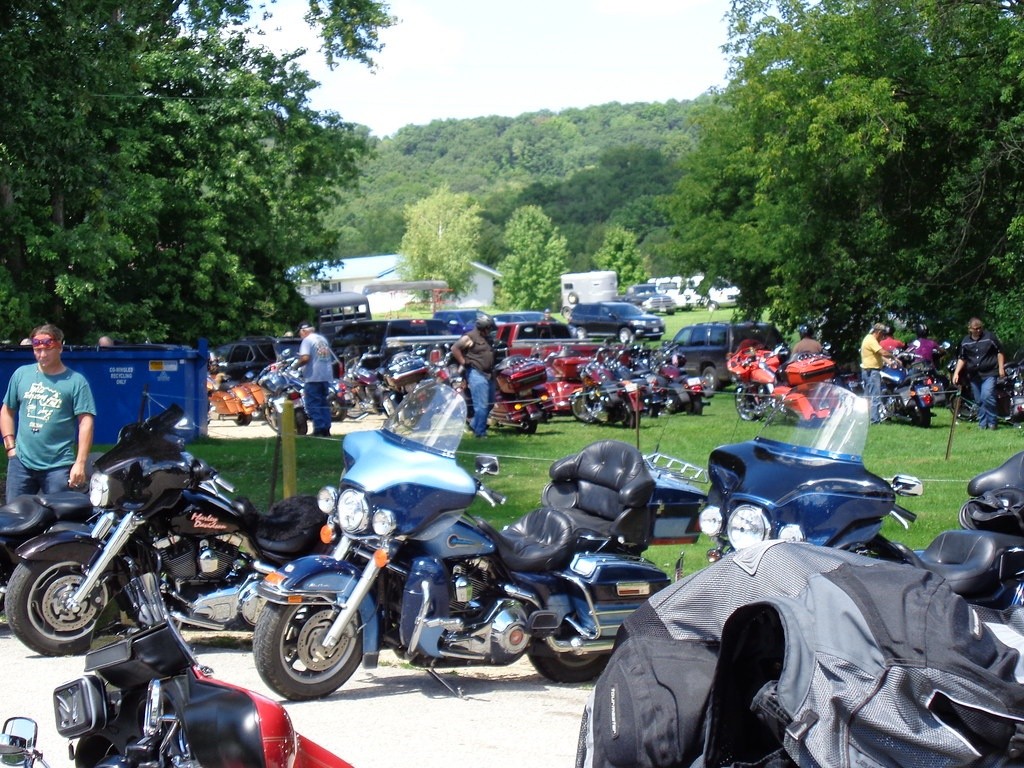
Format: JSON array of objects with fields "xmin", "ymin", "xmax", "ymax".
[{"xmin": 303, "ymin": 291, "xmax": 372, "ymax": 325}]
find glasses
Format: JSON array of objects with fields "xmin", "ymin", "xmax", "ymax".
[
  {"xmin": 971, "ymin": 326, "xmax": 982, "ymax": 331},
  {"xmin": 30, "ymin": 338, "xmax": 56, "ymax": 348}
]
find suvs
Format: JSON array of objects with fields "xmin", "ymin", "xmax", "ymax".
[
  {"xmin": 327, "ymin": 319, "xmax": 465, "ymax": 371},
  {"xmin": 662, "ymin": 322, "xmax": 793, "ymax": 394},
  {"xmin": 210, "ymin": 334, "xmax": 304, "ymax": 383},
  {"xmin": 567, "ymin": 300, "xmax": 666, "ymax": 345}
]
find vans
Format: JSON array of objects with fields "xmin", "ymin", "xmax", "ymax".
[{"xmin": 646, "ymin": 270, "xmax": 741, "ymax": 312}]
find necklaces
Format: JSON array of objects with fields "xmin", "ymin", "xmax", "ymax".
[{"xmin": 31, "ymin": 366, "xmax": 40, "ymax": 433}]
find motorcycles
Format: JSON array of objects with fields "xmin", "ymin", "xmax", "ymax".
[
  {"xmin": 253, "ymin": 381, "xmax": 710, "ymax": 698},
  {"xmin": 0, "ymin": 572, "xmax": 357, "ymax": 768},
  {"xmin": 696, "ymin": 378, "xmax": 1023, "ymax": 621},
  {"xmin": 4, "ymin": 391, "xmax": 343, "ymax": 668},
  {"xmin": 203, "ymin": 325, "xmax": 1023, "ymax": 438}
]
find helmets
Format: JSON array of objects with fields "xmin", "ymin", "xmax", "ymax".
[
  {"xmin": 882, "ymin": 324, "xmax": 896, "ymax": 336},
  {"xmin": 916, "ymin": 323, "xmax": 929, "ymax": 338},
  {"xmin": 476, "ymin": 313, "xmax": 497, "ymax": 331},
  {"xmin": 799, "ymin": 322, "xmax": 816, "ymax": 339}
]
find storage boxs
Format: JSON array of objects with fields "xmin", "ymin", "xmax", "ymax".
[
  {"xmin": 786, "ymin": 358, "xmax": 836, "ymax": 387},
  {"xmin": 570, "ymin": 553, "xmax": 671, "ymax": 634},
  {"xmin": 389, "ymin": 358, "xmax": 429, "ymax": 386},
  {"xmin": 498, "ymin": 361, "xmax": 549, "ymax": 394},
  {"xmin": 879, "ymin": 366, "xmax": 904, "ymax": 384},
  {"xmin": 646, "ymin": 470, "xmax": 708, "ymax": 542},
  {"xmin": 596, "ymin": 393, "xmax": 623, "ymax": 407},
  {"xmin": 700, "ymin": 379, "xmax": 714, "ymax": 397},
  {"xmin": 665, "ymin": 384, "xmax": 691, "ymax": 403}
]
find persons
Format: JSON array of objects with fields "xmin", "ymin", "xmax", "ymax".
[
  {"xmin": 792, "ymin": 324, "xmax": 822, "ymax": 355},
  {"xmin": 0, "ymin": 324, "xmax": 97, "ymax": 506},
  {"xmin": 860, "ymin": 323, "xmax": 908, "ymax": 424},
  {"xmin": 449, "ymin": 311, "xmax": 496, "ymax": 438},
  {"xmin": 906, "ymin": 324, "xmax": 948, "ymax": 365},
  {"xmin": 293, "ymin": 320, "xmax": 333, "ymax": 436},
  {"xmin": 540, "ymin": 308, "xmax": 556, "ymax": 321},
  {"xmin": 99, "ymin": 336, "xmax": 113, "ymax": 346},
  {"xmin": 952, "ymin": 318, "xmax": 1005, "ymax": 428}
]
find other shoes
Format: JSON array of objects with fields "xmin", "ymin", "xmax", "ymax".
[
  {"xmin": 307, "ymin": 430, "xmax": 333, "ymax": 437},
  {"xmin": 464, "ymin": 425, "xmax": 475, "ymax": 437},
  {"xmin": 479, "ymin": 434, "xmax": 488, "ymax": 440},
  {"xmin": 989, "ymin": 424, "xmax": 998, "ymax": 431}
]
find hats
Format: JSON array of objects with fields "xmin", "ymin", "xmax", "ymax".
[
  {"xmin": 872, "ymin": 322, "xmax": 887, "ymax": 331},
  {"xmin": 545, "ymin": 308, "xmax": 551, "ymax": 314},
  {"xmin": 294, "ymin": 320, "xmax": 314, "ymax": 334}
]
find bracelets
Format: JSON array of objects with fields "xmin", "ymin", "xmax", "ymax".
[{"xmin": 3, "ymin": 434, "xmax": 15, "ymax": 452}]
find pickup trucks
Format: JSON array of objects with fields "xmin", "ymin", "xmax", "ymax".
[
  {"xmin": 620, "ymin": 284, "xmax": 678, "ymax": 316},
  {"xmin": 493, "ymin": 323, "xmax": 633, "ymax": 414}
]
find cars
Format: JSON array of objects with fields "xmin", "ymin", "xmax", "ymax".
[{"xmin": 435, "ymin": 309, "xmax": 560, "ymax": 334}]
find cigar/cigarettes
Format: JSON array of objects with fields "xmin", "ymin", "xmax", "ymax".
[{"xmin": 68, "ymin": 479, "xmax": 79, "ymax": 488}]
